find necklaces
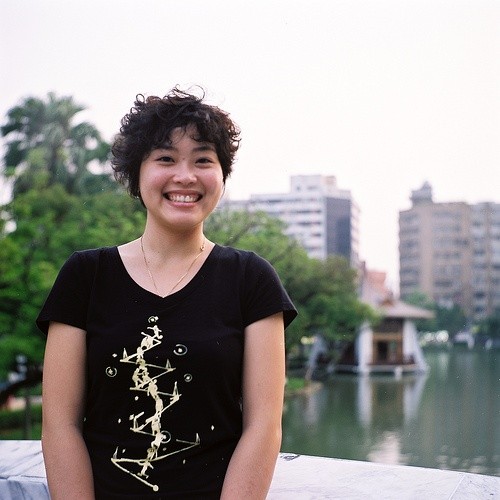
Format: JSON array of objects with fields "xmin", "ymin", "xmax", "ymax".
[{"xmin": 140, "ymin": 236, "xmax": 207, "ymax": 297}]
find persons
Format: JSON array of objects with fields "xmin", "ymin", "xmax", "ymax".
[{"xmin": 35, "ymin": 85, "xmax": 299, "ymax": 500}]
